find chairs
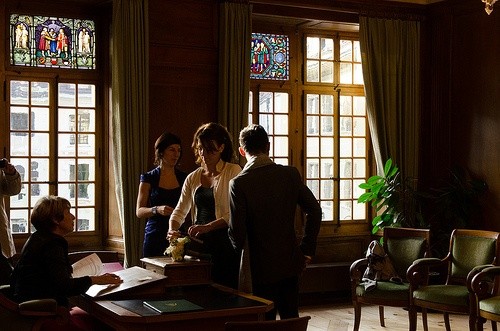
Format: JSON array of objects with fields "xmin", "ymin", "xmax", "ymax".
[
  {"xmin": 0, "ymin": 285, "xmax": 82, "ymax": 331},
  {"xmin": 68, "ymin": 251, "xmax": 118, "ymax": 265},
  {"xmin": 224, "ymin": 316, "xmax": 311, "ymax": 331},
  {"xmin": 348, "ymin": 227, "xmax": 500, "ymax": 331}
]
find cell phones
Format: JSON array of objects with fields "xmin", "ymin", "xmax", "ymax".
[{"xmin": 0, "ymin": 160, "xmax": 6, "ymax": 168}]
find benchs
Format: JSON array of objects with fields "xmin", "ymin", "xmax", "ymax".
[{"xmin": 298, "ymin": 238, "xmax": 366, "ymax": 294}]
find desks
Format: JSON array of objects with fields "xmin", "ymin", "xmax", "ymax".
[{"xmin": 67, "ymin": 279, "xmax": 274, "ymax": 331}]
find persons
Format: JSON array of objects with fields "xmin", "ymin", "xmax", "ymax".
[
  {"xmin": 228, "ymin": 124, "xmax": 322, "ymax": 320},
  {"xmin": 8, "ymin": 195, "xmax": 123, "ymax": 331},
  {"xmin": 166, "ymin": 123, "xmax": 243, "ymax": 290},
  {"xmin": 135, "ymin": 132, "xmax": 192, "ymax": 260},
  {"xmin": 0, "ymin": 158, "xmax": 22, "ymax": 285}
]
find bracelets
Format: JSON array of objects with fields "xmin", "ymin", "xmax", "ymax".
[{"xmin": 152, "ymin": 206, "xmax": 157, "ymax": 216}]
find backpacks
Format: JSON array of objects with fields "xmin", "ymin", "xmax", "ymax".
[{"xmin": 362, "ymin": 239, "xmax": 400, "ymax": 281}]
